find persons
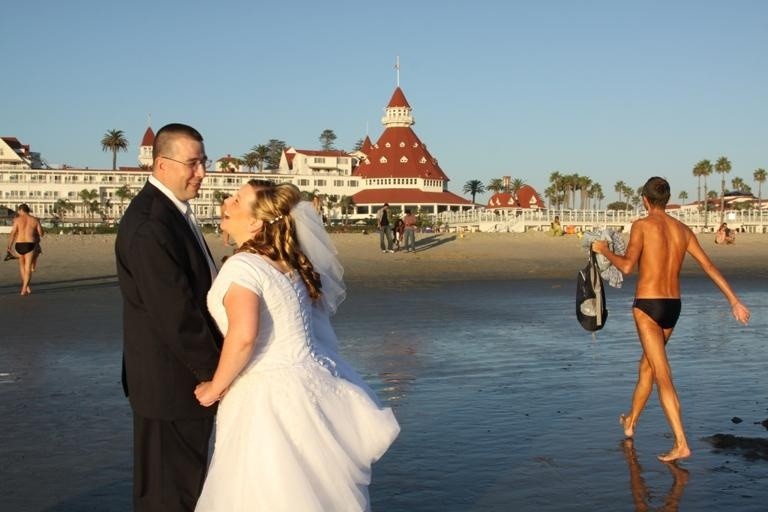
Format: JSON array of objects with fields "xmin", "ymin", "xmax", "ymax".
[
  {"xmin": 375, "ymin": 203, "xmax": 417, "ymax": 254},
  {"xmin": 551, "ymin": 216, "xmax": 564, "ymax": 236},
  {"xmin": 8, "ymin": 203, "xmax": 43, "ymax": 296},
  {"xmin": 591, "ymin": 176, "xmax": 749, "ymax": 463},
  {"xmin": 310, "ymin": 195, "xmax": 323, "ymax": 217},
  {"xmin": 193, "ymin": 180, "xmax": 402, "ymax": 512},
  {"xmin": 715, "ymin": 221, "xmax": 738, "ymax": 247},
  {"xmin": 220, "ymin": 193, "xmax": 232, "ymax": 248},
  {"xmin": 32, "ymin": 238, "xmax": 38, "ymax": 271},
  {"xmin": 114, "ymin": 123, "xmax": 219, "ymax": 512}
]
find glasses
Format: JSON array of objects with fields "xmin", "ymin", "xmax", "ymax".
[{"xmin": 160, "ymin": 156, "xmax": 213, "ymax": 170}]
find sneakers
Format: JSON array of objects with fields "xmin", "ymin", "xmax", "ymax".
[{"xmin": 381, "ymin": 247, "xmax": 416, "ymax": 253}]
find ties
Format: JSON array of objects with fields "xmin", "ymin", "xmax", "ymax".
[{"xmin": 186, "ymin": 205, "xmax": 218, "ymax": 286}]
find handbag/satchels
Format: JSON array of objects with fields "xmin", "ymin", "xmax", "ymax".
[{"xmin": 572, "ymin": 243, "xmax": 610, "ymax": 333}]
